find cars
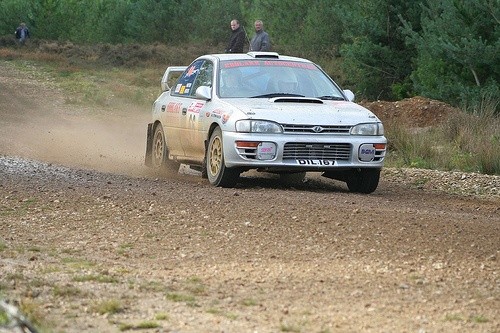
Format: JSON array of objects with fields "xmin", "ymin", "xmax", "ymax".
[{"xmin": 144, "ymin": 25, "xmax": 388, "ymax": 195}]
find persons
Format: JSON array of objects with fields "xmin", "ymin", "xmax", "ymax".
[
  {"xmin": 273, "ymin": 68, "xmax": 302, "ymax": 96},
  {"xmin": 220, "ymin": 67, "xmax": 264, "ymax": 97},
  {"xmin": 223, "ymin": 19, "xmax": 246, "ymax": 54},
  {"xmin": 248, "ymin": 19, "xmax": 272, "ymax": 53},
  {"xmin": 15, "ymin": 23, "xmax": 31, "ymax": 51}
]
